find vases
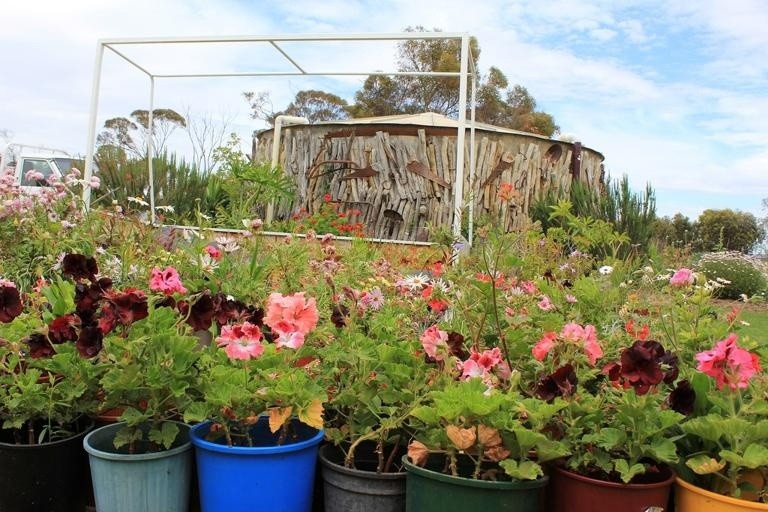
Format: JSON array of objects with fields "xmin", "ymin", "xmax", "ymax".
[
  {"xmin": 674, "ymin": 458, "xmax": 768, "ymax": 511},
  {"xmin": 319, "ymin": 445, "xmax": 402, "ymax": 508},
  {"xmin": 0, "ymin": 427, "xmax": 82, "ymax": 510},
  {"xmin": 402, "ymin": 451, "xmax": 549, "ymax": 511},
  {"xmin": 553, "ymin": 457, "xmax": 673, "ymax": 511},
  {"xmin": 82, "ymin": 417, "xmax": 188, "ymax": 509}
]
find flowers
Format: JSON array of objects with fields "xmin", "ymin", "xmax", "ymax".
[{"xmin": 1, "ymin": 167, "xmax": 767, "ymax": 407}]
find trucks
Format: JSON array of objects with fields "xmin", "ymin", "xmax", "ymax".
[{"xmin": 1, "ymin": 143, "xmax": 85, "ymax": 197}]
